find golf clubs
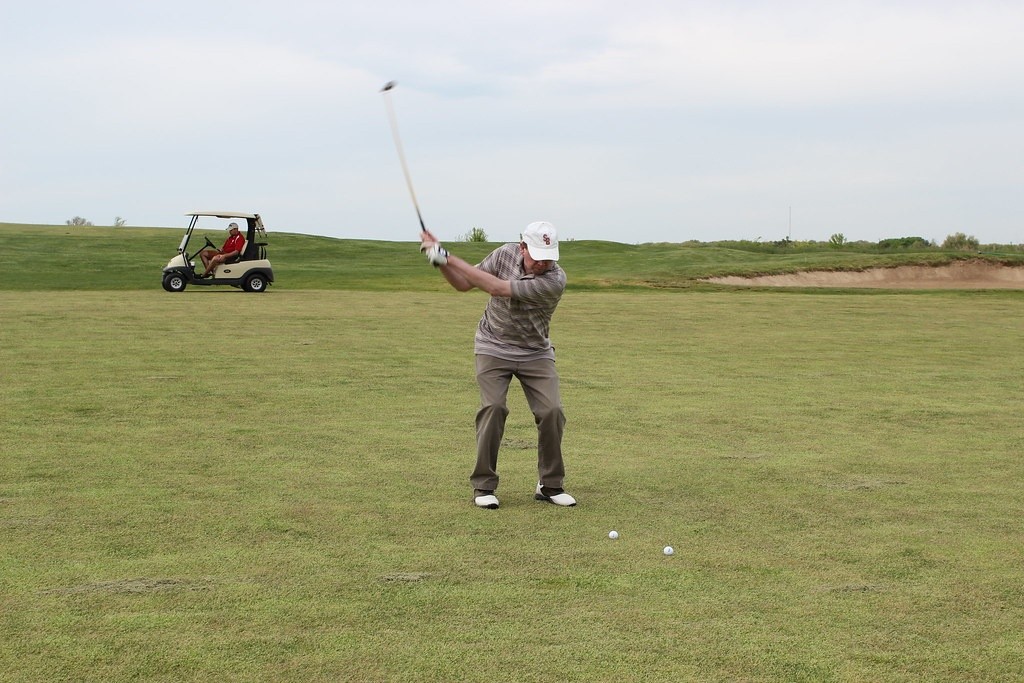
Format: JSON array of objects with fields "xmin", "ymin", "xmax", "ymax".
[{"xmin": 379, "ymin": 79, "xmax": 442, "ymax": 269}]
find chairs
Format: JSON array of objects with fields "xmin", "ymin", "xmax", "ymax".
[{"xmin": 225, "ymin": 239, "xmax": 249, "ymax": 265}]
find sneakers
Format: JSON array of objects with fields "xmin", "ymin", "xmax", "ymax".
[
  {"xmin": 473, "ymin": 488, "xmax": 499, "ymax": 509},
  {"xmin": 535, "ymin": 481, "xmax": 577, "ymax": 506}
]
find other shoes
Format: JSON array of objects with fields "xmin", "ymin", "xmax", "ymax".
[
  {"xmin": 195, "ymin": 274, "xmax": 205, "ymax": 279},
  {"xmin": 205, "ymin": 271, "xmax": 213, "ymax": 278}
]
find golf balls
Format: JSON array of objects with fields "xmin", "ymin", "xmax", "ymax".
[
  {"xmin": 608, "ymin": 530, "xmax": 619, "ymax": 539},
  {"xmin": 663, "ymin": 546, "xmax": 674, "ymax": 555}
]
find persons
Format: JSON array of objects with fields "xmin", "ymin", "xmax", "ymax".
[
  {"xmin": 195, "ymin": 223, "xmax": 245, "ymax": 279},
  {"xmin": 419, "ymin": 221, "xmax": 576, "ymax": 509}
]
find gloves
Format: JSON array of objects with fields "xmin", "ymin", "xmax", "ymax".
[{"xmin": 419, "ymin": 241, "xmax": 449, "ymax": 267}]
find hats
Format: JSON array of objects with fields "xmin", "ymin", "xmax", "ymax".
[
  {"xmin": 226, "ymin": 223, "xmax": 238, "ymax": 230},
  {"xmin": 522, "ymin": 222, "xmax": 560, "ymax": 261}
]
